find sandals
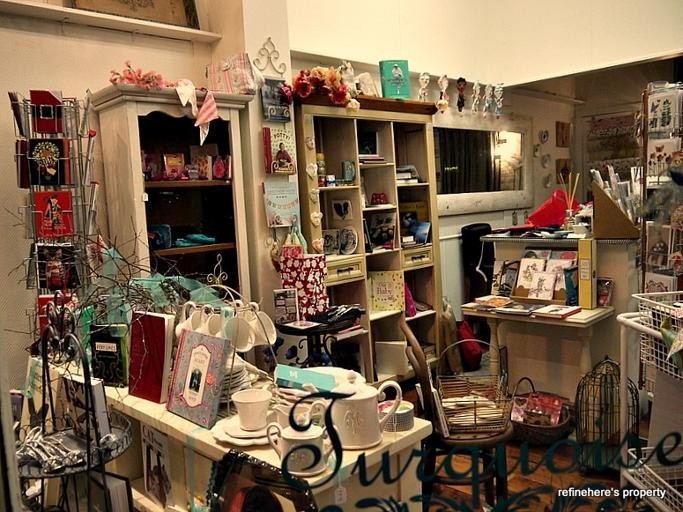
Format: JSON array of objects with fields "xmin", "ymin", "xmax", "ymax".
[{"xmin": 13, "ymin": 421, "xmax": 87, "ymax": 472}]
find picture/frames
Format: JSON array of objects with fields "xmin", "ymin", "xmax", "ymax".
[{"xmin": 72, "ymin": 0, "xmax": 200, "ymax": 30}]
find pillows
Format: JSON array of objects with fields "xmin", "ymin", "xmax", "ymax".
[
  {"xmin": 457, "ymin": 320, "xmax": 483, "ymax": 371},
  {"xmin": 441, "ymin": 295, "xmax": 464, "ymax": 375}
]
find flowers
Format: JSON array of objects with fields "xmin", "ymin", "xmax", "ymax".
[
  {"xmin": 108, "ymin": 59, "xmax": 175, "ymax": 91},
  {"xmin": 278, "ymin": 66, "xmax": 361, "ymax": 115}
]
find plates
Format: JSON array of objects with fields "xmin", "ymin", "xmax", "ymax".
[
  {"xmin": 544, "ymin": 172, "xmax": 554, "ymax": 189},
  {"xmin": 279, "ymin": 363, "xmax": 366, "ymax": 396},
  {"xmin": 216, "ymin": 356, "xmax": 253, "ymax": 405},
  {"xmin": 534, "ymin": 143, "xmax": 541, "ymax": 158},
  {"xmin": 274, "ymin": 388, "xmax": 329, "ymax": 404},
  {"xmin": 540, "ymin": 128, "xmax": 549, "ymax": 144},
  {"xmin": 541, "ymin": 152, "xmax": 551, "ymax": 169},
  {"xmin": 210, "ymin": 413, "xmax": 282, "ymax": 446}
]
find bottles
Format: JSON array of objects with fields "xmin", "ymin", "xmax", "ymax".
[{"xmin": 316, "ymin": 153, "xmax": 327, "ymax": 187}]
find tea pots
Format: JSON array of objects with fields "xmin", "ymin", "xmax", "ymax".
[{"xmin": 267, "ymin": 371, "xmax": 405, "ymax": 479}]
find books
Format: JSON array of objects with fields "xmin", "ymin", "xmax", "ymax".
[
  {"xmin": 359, "ymin": 154, "xmax": 388, "ymax": 164},
  {"xmin": 401, "ymin": 221, "xmax": 431, "ymax": 248},
  {"xmin": 141, "ymin": 143, "xmax": 232, "ymax": 181},
  {"xmin": 7, "ymin": 88, "xmax": 83, "ymax": 340},
  {"xmin": 63, "ymin": 372, "xmax": 113, "ymax": 445},
  {"xmin": 88, "ymin": 468, "xmax": 134, "ymax": 512},
  {"xmin": 460, "ymin": 89, "xmax": 683, "ymax": 322},
  {"xmin": 404, "ymin": 282, "xmax": 433, "ymax": 318},
  {"xmin": 165, "ymin": 329, "xmax": 232, "ymax": 431},
  {"xmin": 89, "ymin": 322, "xmax": 130, "ymax": 389},
  {"xmin": 397, "ymin": 169, "xmax": 420, "ymax": 183},
  {"xmin": 363, "ymin": 218, "xmax": 373, "ymax": 253},
  {"xmin": 272, "ymin": 288, "xmax": 300, "ymax": 323},
  {"xmin": 379, "ymin": 59, "xmax": 411, "ymax": 100},
  {"xmin": 128, "ymin": 310, "xmax": 176, "ymax": 404},
  {"xmin": 369, "ymin": 212, "xmax": 396, "ymax": 250}
]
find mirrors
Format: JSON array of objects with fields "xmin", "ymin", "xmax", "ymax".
[{"xmin": 432, "ymin": 107, "xmax": 534, "ymax": 216}]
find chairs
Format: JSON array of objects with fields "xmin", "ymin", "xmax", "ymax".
[{"xmin": 399, "ymin": 321, "xmax": 513, "ymax": 512}]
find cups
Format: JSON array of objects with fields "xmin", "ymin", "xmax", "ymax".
[
  {"xmin": 230, "ymin": 387, "xmax": 273, "ymax": 431},
  {"xmin": 174, "ymin": 298, "xmax": 277, "ymax": 356},
  {"xmin": 374, "ymin": 397, "xmax": 415, "ymax": 433}
]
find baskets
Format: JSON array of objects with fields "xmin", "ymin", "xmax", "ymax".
[
  {"xmin": 627, "ymin": 444, "xmax": 683, "ymax": 512},
  {"xmin": 437, "ymin": 340, "xmax": 516, "ymax": 433},
  {"xmin": 513, "ymin": 377, "xmax": 571, "ymax": 445},
  {"xmin": 632, "ymin": 290, "xmax": 683, "ymax": 380}
]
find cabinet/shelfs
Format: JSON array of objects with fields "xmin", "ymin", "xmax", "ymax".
[
  {"xmin": 16, "ymin": 290, "xmax": 133, "ymax": 512},
  {"xmin": 91, "ymin": 83, "xmax": 257, "ymax": 369},
  {"xmin": 295, "ymin": 103, "xmax": 446, "ymax": 400},
  {"xmin": 19, "ymin": 353, "xmax": 432, "ymax": 512},
  {"xmin": 616, "ymin": 83, "xmax": 683, "ymax": 512}
]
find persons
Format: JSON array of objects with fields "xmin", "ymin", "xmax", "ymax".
[
  {"xmin": 391, "ymin": 64, "xmax": 403, "ymax": 95},
  {"xmin": 276, "ymin": 142, "xmax": 292, "ymax": 169}
]
